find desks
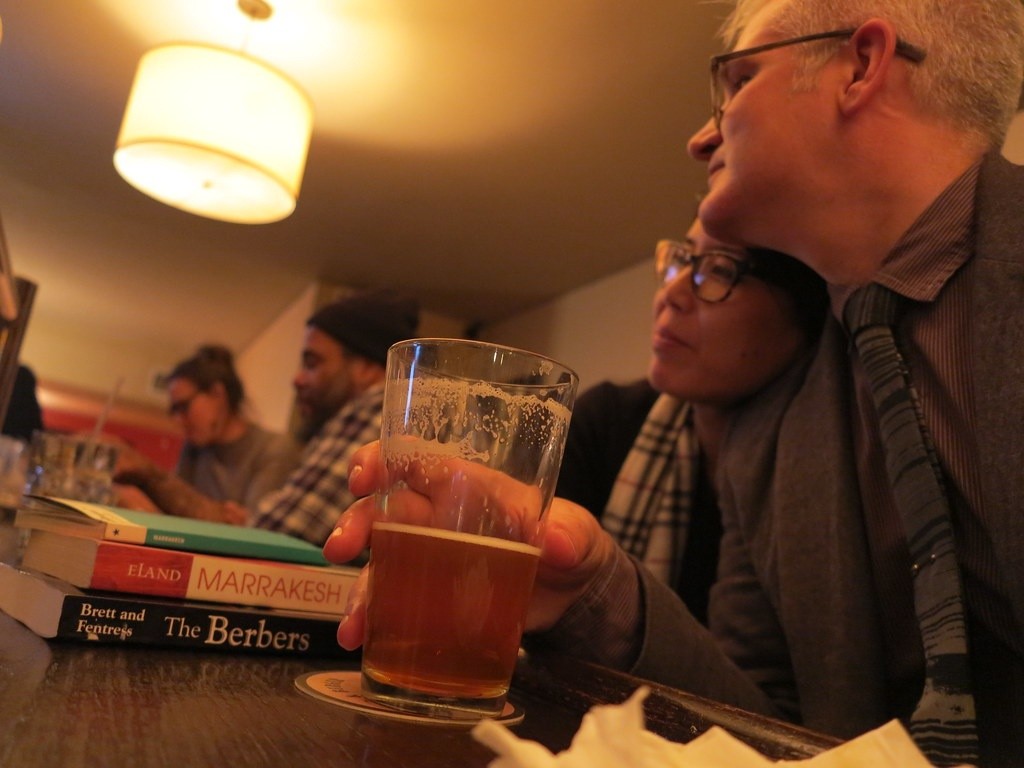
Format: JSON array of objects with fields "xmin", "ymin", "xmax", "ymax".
[{"xmin": 0, "ymin": 513, "xmax": 849, "ymax": 767}]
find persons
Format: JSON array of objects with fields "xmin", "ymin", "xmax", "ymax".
[
  {"xmin": 123, "ymin": 290, "xmax": 423, "ymax": 544},
  {"xmin": 320, "ymin": 0, "xmax": 1024, "ymax": 768},
  {"xmin": 149, "ymin": 341, "xmax": 295, "ymax": 518},
  {"xmin": 514, "ymin": 210, "xmax": 831, "ymax": 634}
]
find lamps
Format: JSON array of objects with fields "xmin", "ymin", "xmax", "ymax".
[{"xmin": 112, "ymin": 0, "xmax": 317, "ymax": 226}]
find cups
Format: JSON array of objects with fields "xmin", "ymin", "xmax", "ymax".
[
  {"xmin": 21, "ymin": 429, "xmax": 118, "ymax": 505},
  {"xmin": 361, "ymin": 338, "xmax": 581, "ymax": 724}
]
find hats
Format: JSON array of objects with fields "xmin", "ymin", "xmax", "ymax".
[
  {"xmin": 308, "ymin": 286, "xmax": 420, "ymax": 368},
  {"xmin": 171, "ymin": 345, "xmax": 238, "ymax": 390}
]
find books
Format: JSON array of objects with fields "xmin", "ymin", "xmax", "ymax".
[{"xmin": 0, "ymin": 491, "xmax": 367, "ymax": 667}]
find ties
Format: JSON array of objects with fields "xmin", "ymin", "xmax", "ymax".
[{"xmin": 844, "ymin": 284, "xmax": 982, "ymax": 767}]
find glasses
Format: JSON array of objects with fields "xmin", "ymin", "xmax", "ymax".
[
  {"xmin": 653, "ymin": 236, "xmax": 786, "ymax": 303},
  {"xmin": 709, "ymin": 26, "xmax": 926, "ymax": 103},
  {"xmin": 168, "ymin": 391, "xmax": 205, "ymax": 412}
]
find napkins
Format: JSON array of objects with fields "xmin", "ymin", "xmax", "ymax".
[{"xmin": 472, "ymin": 695, "xmax": 935, "ymax": 768}]
